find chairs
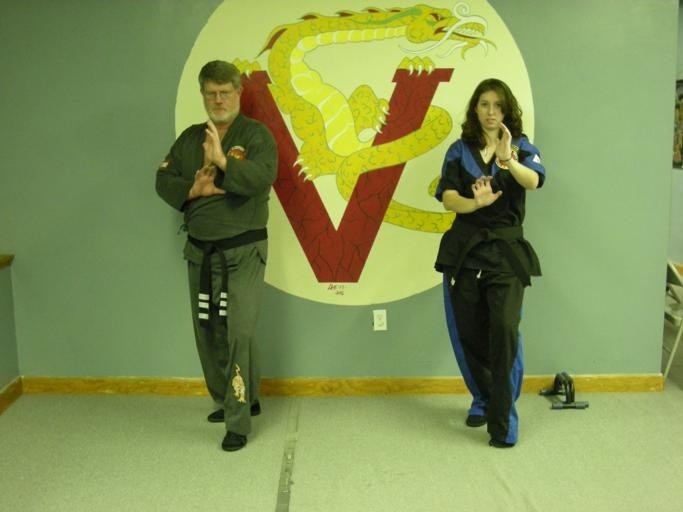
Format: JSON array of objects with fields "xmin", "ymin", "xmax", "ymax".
[{"xmin": 663, "ymin": 260, "xmax": 683, "ymax": 379}]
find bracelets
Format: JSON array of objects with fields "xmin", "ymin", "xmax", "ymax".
[{"xmin": 499, "ymin": 154, "xmax": 513, "ymax": 163}]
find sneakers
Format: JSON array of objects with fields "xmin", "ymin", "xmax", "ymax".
[
  {"xmin": 466, "ymin": 414, "xmax": 487, "ymax": 427},
  {"xmin": 208, "ymin": 400, "xmax": 260, "ymax": 450}
]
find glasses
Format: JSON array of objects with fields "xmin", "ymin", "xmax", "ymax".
[{"xmin": 202, "ymin": 87, "xmax": 239, "ymax": 100}]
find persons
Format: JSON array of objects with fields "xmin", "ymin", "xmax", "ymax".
[
  {"xmin": 434, "ymin": 78, "xmax": 547, "ymax": 447},
  {"xmin": 155, "ymin": 60, "xmax": 278, "ymax": 453}
]
